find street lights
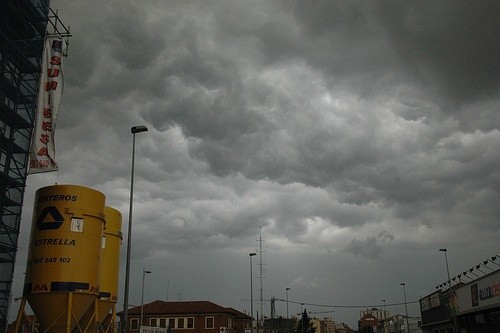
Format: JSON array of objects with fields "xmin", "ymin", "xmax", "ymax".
[
  {"xmin": 382, "ymin": 300, "xmax": 388, "ymax": 333},
  {"xmin": 400, "ymin": 283, "xmax": 410, "ymax": 333},
  {"xmin": 121, "ymin": 125, "xmax": 148, "ymax": 333},
  {"xmin": 301, "ymin": 303, "xmax": 305, "ymax": 333},
  {"xmin": 140, "ymin": 267, "xmax": 151, "ymax": 326},
  {"xmin": 286, "ymin": 287, "xmax": 290, "ymax": 333},
  {"xmin": 439, "ymin": 248, "xmax": 458, "ymax": 333},
  {"xmin": 250, "ymin": 253, "xmax": 256, "ymax": 333}
]
